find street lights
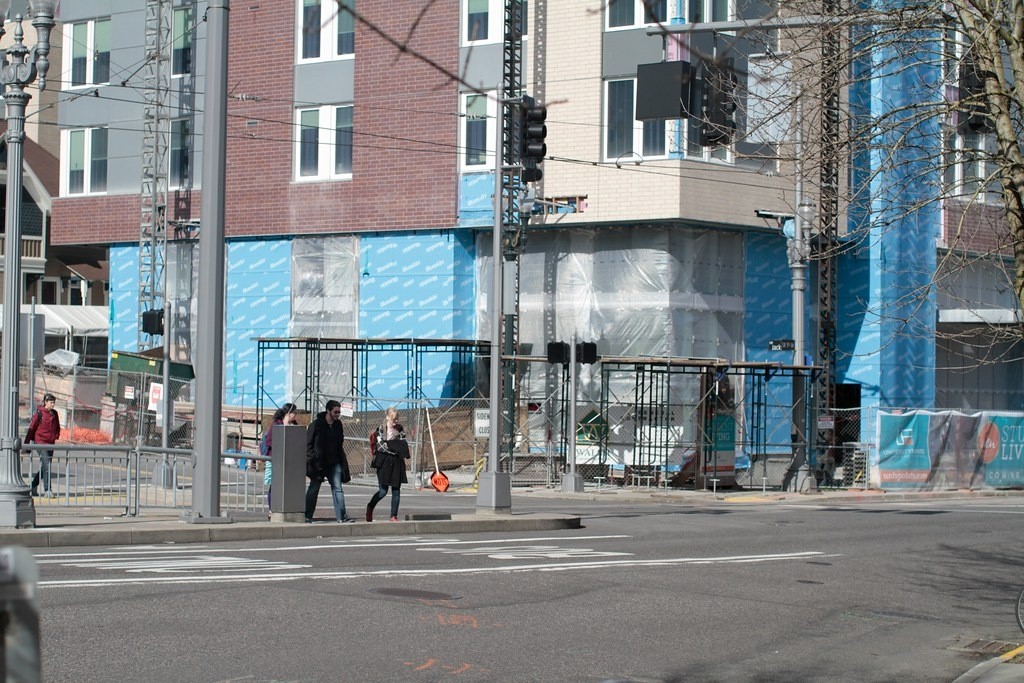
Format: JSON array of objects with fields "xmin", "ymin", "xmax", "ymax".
[
  {"xmin": 491, "ymin": 188, "xmax": 527, "ymax": 460},
  {"xmin": 0, "ymin": 0, "xmax": 64, "ymax": 525},
  {"xmin": 783, "ymin": 197, "xmax": 817, "ymax": 493}
]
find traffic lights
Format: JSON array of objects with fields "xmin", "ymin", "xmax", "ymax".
[
  {"xmin": 142, "ymin": 311, "xmax": 163, "ymax": 335},
  {"xmin": 578, "ymin": 342, "xmax": 596, "ymax": 365},
  {"xmin": 548, "ymin": 341, "xmax": 570, "ymax": 364},
  {"xmin": 521, "ymin": 94, "xmax": 547, "ymax": 159},
  {"xmin": 957, "ymin": 59, "xmax": 994, "ymax": 134},
  {"xmin": 701, "ymin": 58, "xmax": 737, "ymax": 145}
]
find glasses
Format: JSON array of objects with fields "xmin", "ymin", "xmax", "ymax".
[{"xmin": 331, "ymin": 410, "xmax": 341, "ymax": 414}]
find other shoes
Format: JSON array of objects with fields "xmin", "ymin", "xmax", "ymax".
[
  {"xmin": 338, "ymin": 516, "xmax": 355, "ymax": 523},
  {"xmin": 366, "ymin": 503, "xmax": 372, "ymax": 522},
  {"xmin": 268, "ymin": 512, "xmax": 271, "ymax": 519},
  {"xmin": 44, "ymin": 490, "xmax": 55, "ymax": 498},
  {"xmin": 305, "ymin": 518, "xmax": 312, "ymax": 524},
  {"xmin": 29, "ymin": 482, "xmax": 39, "ymax": 497},
  {"xmin": 391, "ymin": 516, "xmax": 398, "ymax": 522}
]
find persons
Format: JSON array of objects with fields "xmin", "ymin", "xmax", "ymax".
[
  {"xmin": 264, "ymin": 403, "xmax": 298, "ymax": 520},
  {"xmin": 21, "ymin": 394, "xmax": 61, "ymax": 498},
  {"xmin": 366, "ymin": 407, "xmax": 410, "ymax": 523},
  {"xmin": 305, "ymin": 400, "xmax": 356, "ymax": 523}
]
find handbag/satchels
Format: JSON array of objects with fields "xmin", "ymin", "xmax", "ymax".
[
  {"xmin": 260, "ymin": 420, "xmax": 281, "ymax": 462},
  {"xmin": 370, "ymin": 423, "xmax": 386, "ymax": 456}
]
